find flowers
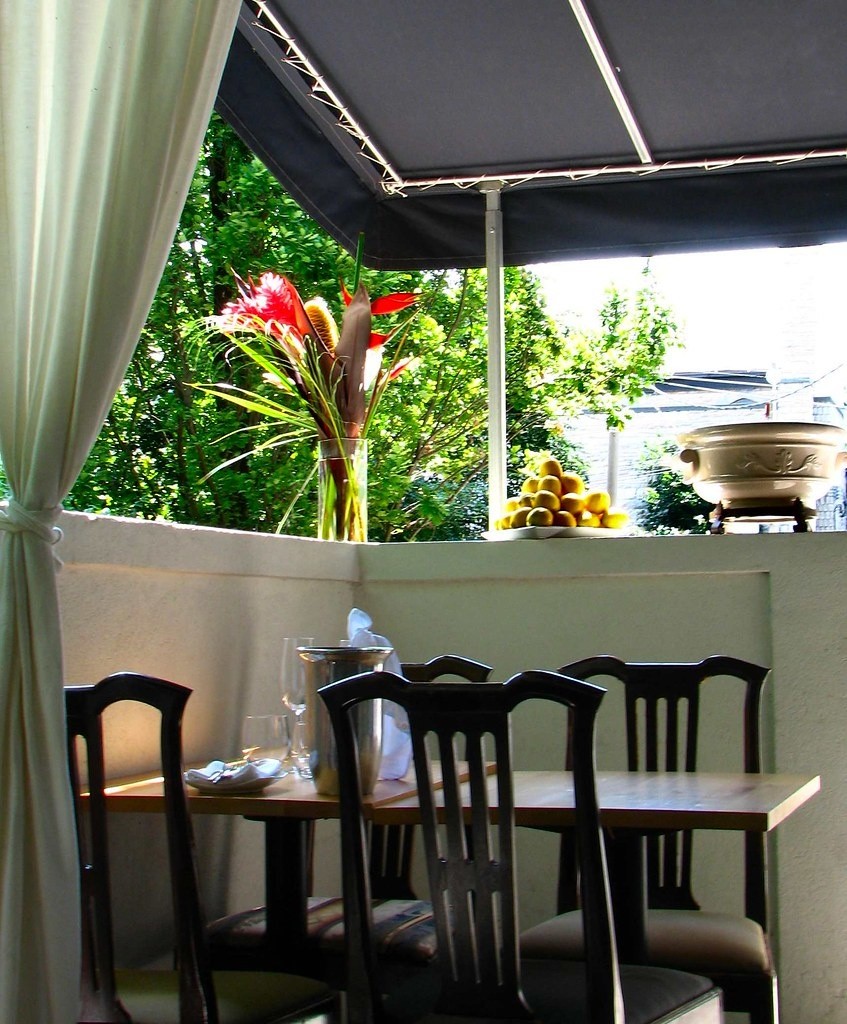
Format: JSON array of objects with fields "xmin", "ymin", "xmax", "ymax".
[{"xmin": 187, "ymin": 228, "xmax": 422, "ymax": 539}]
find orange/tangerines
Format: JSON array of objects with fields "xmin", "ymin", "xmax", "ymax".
[{"xmin": 492, "ymin": 460, "xmax": 625, "ymax": 527}]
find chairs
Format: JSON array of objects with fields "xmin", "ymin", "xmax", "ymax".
[
  {"xmin": 500, "ymin": 656, "xmax": 782, "ymax": 1024},
  {"xmin": 203, "ymin": 653, "xmax": 492, "ymax": 1022},
  {"xmin": 61, "ymin": 670, "xmax": 217, "ymax": 1023},
  {"xmin": 315, "ymin": 670, "xmax": 725, "ymax": 1023}
]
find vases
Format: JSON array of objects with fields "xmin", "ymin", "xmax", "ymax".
[{"xmin": 308, "ymin": 434, "xmax": 372, "ymax": 543}]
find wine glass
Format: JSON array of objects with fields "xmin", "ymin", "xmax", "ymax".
[{"xmin": 280, "ymin": 664, "xmax": 308, "ymax": 774}]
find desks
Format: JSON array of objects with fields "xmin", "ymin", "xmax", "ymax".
[{"xmin": 70, "ymin": 752, "xmax": 821, "ymax": 961}]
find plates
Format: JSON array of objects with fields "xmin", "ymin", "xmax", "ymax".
[
  {"xmin": 479, "ymin": 526, "xmax": 628, "ymax": 539},
  {"xmin": 183, "ymin": 760, "xmax": 288, "ymax": 793}
]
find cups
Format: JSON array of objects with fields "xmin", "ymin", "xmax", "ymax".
[
  {"xmin": 293, "ymin": 720, "xmax": 313, "ymax": 779},
  {"xmin": 242, "ymin": 715, "xmax": 291, "ymax": 763},
  {"xmin": 297, "ymin": 645, "xmax": 394, "ymax": 795}
]
